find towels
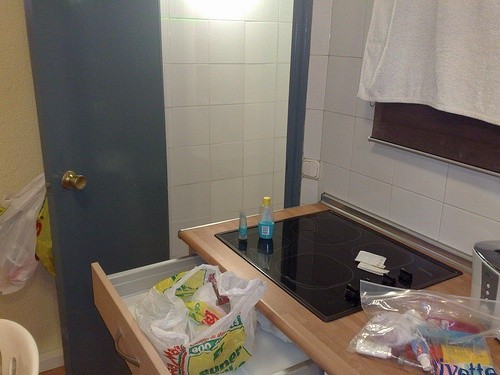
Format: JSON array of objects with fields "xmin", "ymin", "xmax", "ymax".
[
  {"xmin": 438, "ymin": 318, "xmax": 496, "ymax": 375},
  {"xmin": 357, "ymin": 1, "xmax": 500, "ymax": 126}
]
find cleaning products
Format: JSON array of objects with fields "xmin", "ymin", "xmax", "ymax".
[
  {"xmin": 258, "ymin": 196, "xmax": 275, "ymax": 240},
  {"xmin": 238, "ymin": 211, "xmax": 248, "ymax": 240}
]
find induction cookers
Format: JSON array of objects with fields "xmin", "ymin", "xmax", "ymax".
[{"xmin": 215, "ymin": 210, "xmax": 463, "ymax": 322}]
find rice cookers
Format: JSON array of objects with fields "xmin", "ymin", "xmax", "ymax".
[{"xmin": 470, "ymin": 240, "xmax": 500, "ymax": 340}]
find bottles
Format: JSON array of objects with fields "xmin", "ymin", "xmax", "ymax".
[
  {"xmin": 356, "ymin": 329, "xmax": 480, "ymax": 372},
  {"xmin": 258, "ymin": 196, "xmax": 275, "ymax": 239},
  {"xmin": 257, "ymin": 238, "xmax": 273, "ymax": 272},
  {"xmin": 239, "ymin": 211, "xmax": 248, "ymax": 241},
  {"xmin": 238, "ymin": 240, "xmax": 247, "ymax": 255}
]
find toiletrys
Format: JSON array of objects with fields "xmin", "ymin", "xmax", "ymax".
[
  {"xmin": 356, "ymin": 339, "xmax": 392, "ymax": 359},
  {"xmin": 411, "ymin": 336, "xmax": 433, "ymax": 372}
]
